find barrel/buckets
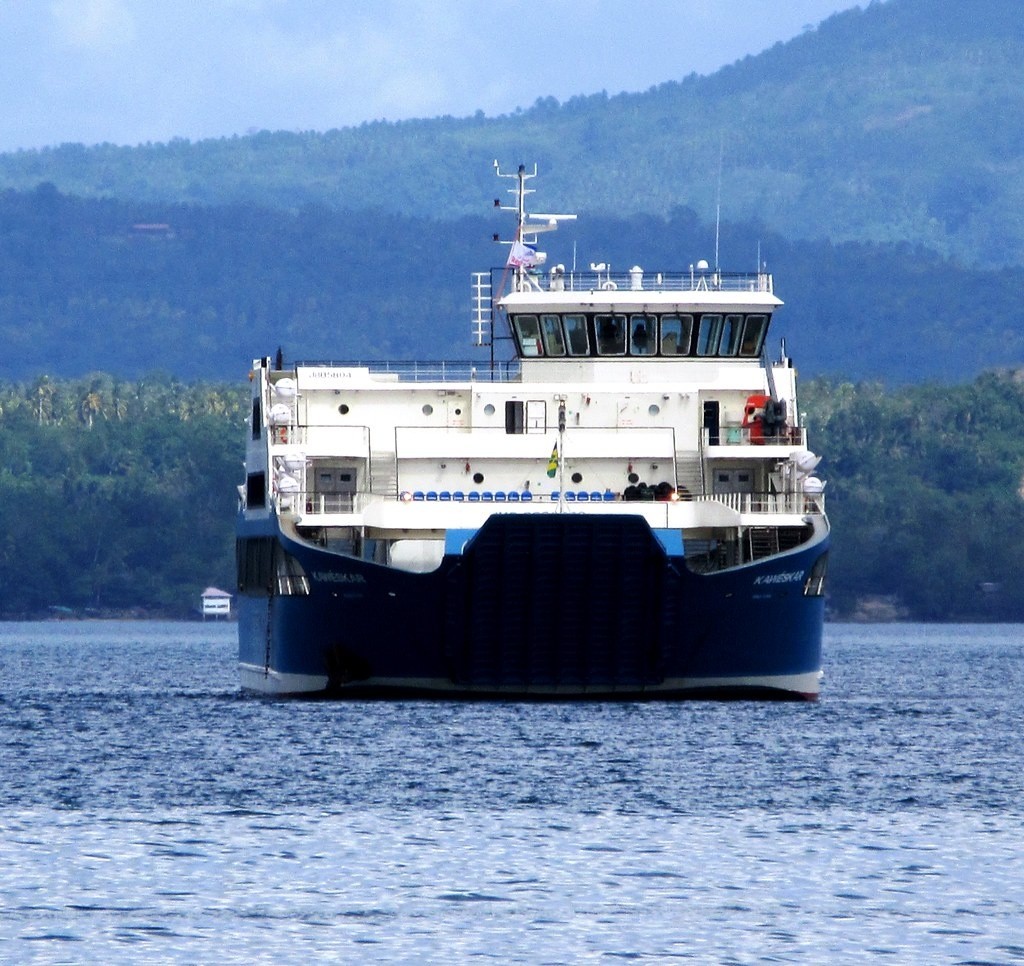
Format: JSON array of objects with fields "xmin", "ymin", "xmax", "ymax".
[{"xmin": 728, "ymin": 421, "xmax": 742, "ymax": 445}]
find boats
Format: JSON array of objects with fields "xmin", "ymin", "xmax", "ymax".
[{"xmin": 232, "ymin": 130, "xmax": 834, "ymax": 708}]
